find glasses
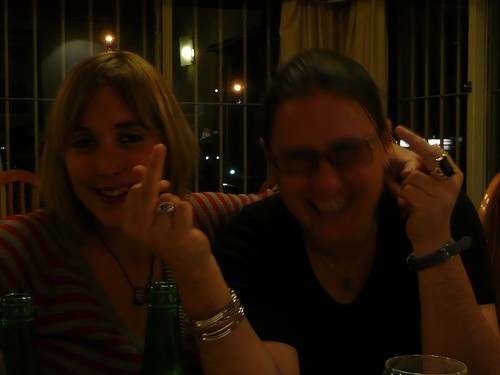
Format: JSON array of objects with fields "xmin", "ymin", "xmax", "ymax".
[{"xmin": 275, "ymin": 136, "xmax": 377, "ymax": 177}]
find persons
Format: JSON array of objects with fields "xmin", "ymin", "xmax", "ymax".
[
  {"xmin": 0, "ymin": 52, "xmax": 421, "ymax": 375},
  {"xmin": 120, "ymin": 49, "xmax": 500, "ymax": 375}
]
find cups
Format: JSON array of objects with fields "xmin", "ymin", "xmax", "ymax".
[{"xmin": 383, "ymin": 353, "xmax": 470, "ymax": 375}]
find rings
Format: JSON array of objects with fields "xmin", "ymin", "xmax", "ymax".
[
  {"xmin": 432, "ymin": 154, "xmax": 457, "ymax": 180},
  {"xmin": 154, "ymin": 199, "xmax": 176, "ymax": 216}
]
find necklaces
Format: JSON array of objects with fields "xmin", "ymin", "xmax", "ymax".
[
  {"xmin": 85, "ymin": 216, "xmax": 157, "ymax": 307},
  {"xmin": 313, "ymin": 247, "xmax": 367, "ymax": 295}
]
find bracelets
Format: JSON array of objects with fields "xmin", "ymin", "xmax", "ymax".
[
  {"xmin": 190, "ymin": 288, "xmax": 245, "ymax": 343},
  {"xmin": 405, "ymin": 234, "xmax": 474, "ymax": 273}
]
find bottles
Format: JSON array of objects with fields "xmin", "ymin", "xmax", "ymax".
[
  {"xmin": 1, "ymin": 292, "xmax": 37, "ymax": 375},
  {"xmin": 140, "ymin": 281, "xmax": 184, "ymax": 375}
]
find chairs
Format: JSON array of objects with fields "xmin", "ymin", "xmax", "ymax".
[{"xmin": 0, "ymin": 170, "xmax": 44, "ymax": 218}]
